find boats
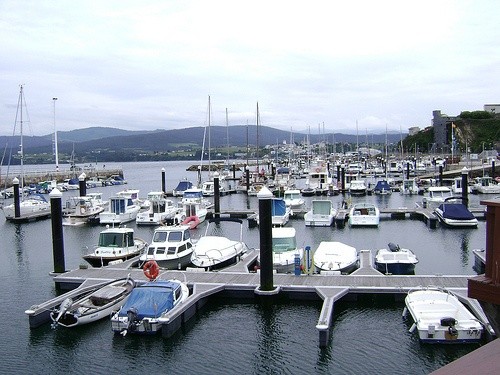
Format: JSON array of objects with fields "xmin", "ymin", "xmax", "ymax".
[
  {"xmin": 159, "ymin": 199, "xmax": 209, "ymax": 230},
  {"xmin": 401, "ymin": 284, "xmax": 496, "ymax": 345},
  {"xmin": 189, "ymin": 221, "xmax": 250, "ymax": 271},
  {"xmin": 346, "ymin": 202, "xmax": 381, "ymax": 229},
  {"xmin": 313, "ymin": 241, "xmax": 360, "ymax": 274},
  {"xmin": 135, "ymin": 167, "xmax": 175, "ymax": 226},
  {"xmin": 303, "ymin": 199, "xmax": 338, "ymax": 227},
  {"xmin": 253, "ymin": 197, "xmax": 294, "ymax": 227},
  {"xmin": 49, "ymin": 272, "xmax": 136, "ymax": 331},
  {"xmin": 109, "ymin": 260, "xmax": 190, "ymax": 338},
  {"xmin": 138, "ymin": 223, "xmax": 198, "ymax": 270},
  {"xmin": 256, "ymin": 223, "xmax": 304, "ymax": 274},
  {"xmin": 81, "ymin": 218, "xmax": 150, "ymax": 267},
  {"xmin": 374, "ymin": 242, "xmax": 420, "ymax": 275},
  {"xmin": 430, "ymin": 196, "xmax": 479, "ymax": 229},
  {"xmin": 99, "ymin": 188, "xmax": 143, "ymax": 225}
]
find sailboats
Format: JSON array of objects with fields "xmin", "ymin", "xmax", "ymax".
[
  {"xmin": 0, "ymin": 83, "xmax": 129, "ymax": 225},
  {"xmin": 172, "ymin": 92, "xmax": 500, "ymax": 210}
]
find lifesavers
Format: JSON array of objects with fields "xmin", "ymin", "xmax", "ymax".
[
  {"xmin": 316, "ymin": 168, "xmax": 320, "ymax": 173},
  {"xmin": 143, "ymin": 260, "xmax": 159, "ymax": 279}
]
